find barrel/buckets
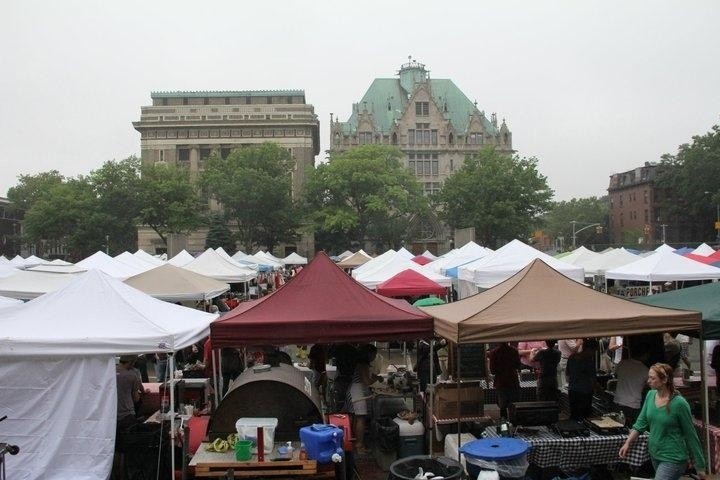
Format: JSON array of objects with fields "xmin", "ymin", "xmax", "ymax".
[
  {"xmin": 301, "ymin": 423, "xmax": 346, "ymax": 464},
  {"xmin": 393, "ymin": 412, "xmax": 424, "ymax": 455},
  {"xmin": 234, "ymin": 440, "xmax": 253, "ymax": 460}
]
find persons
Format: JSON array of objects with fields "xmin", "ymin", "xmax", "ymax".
[
  {"xmin": 203, "ymin": 263, "xmax": 303, "ymax": 312},
  {"xmin": 617, "ymin": 361, "xmax": 707, "ymax": 480},
  {"xmin": 113, "ymin": 329, "xmax": 719, "ymax": 456}
]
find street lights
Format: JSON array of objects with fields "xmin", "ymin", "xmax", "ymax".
[
  {"xmin": 660, "ymin": 223, "xmax": 670, "ymax": 244},
  {"xmin": 104, "ymin": 233, "xmax": 110, "ymax": 255},
  {"xmin": 11, "ymin": 222, "xmax": 18, "ymax": 233},
  {"xmin": 705, "ymin": 191, "xmax": 720, "ymax": 241},
  {"xmin": 571, "ymin": 219, "xmax": 601, "ymax": 249}
]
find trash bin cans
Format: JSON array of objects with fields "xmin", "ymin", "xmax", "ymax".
[
  {"xmin": 373, "ymin": 415, "xmax": 425, "ymax": 472},
  {"xmin": 390, "ymin": 437, "xmax": 532, "ymax": 480}
]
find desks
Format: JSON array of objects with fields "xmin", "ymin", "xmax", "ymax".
[{"xmin": 141, "ymin": 363, "xmax": 720, "ymax": 480}]
